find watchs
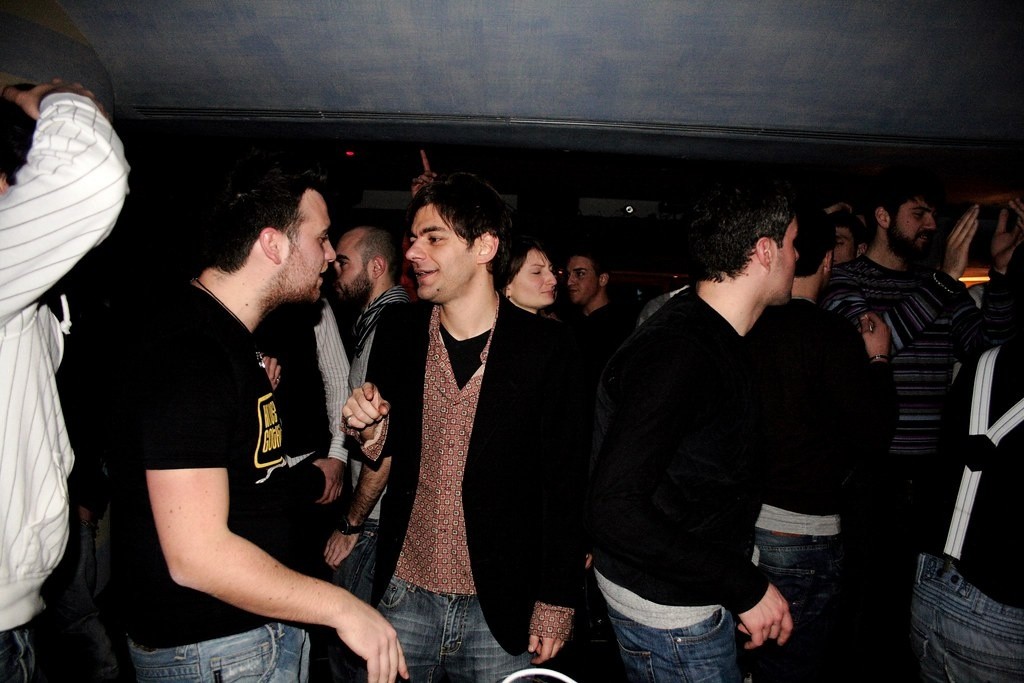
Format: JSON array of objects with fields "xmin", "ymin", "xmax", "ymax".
[{"xmin": 336, "ymin": 515, "xmax": 362, "ymax": 535}]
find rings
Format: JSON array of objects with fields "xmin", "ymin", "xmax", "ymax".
[
  {"xmin": 276, "ymin": 378, "xmax": 280, "ymax": 385},
  {"xmin": 344, "ymin": 414, "xmax": 355, "ymax": 429}
]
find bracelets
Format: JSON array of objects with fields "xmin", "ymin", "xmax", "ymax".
[
  {"xmin": 869, "ymin": 354, "xmax": 889, "ymax": 362},
  {"xmin": 1, "ymin": 85, "xmax": 15, "ymax": 96},
  {"xmin": 933, "ymin": 272, "xmax": 961, "ymax": 295}
]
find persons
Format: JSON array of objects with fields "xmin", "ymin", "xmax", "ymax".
[{"xmin": 0, "ymin": 77, "xmax": 1024, "ymax": 683}]
